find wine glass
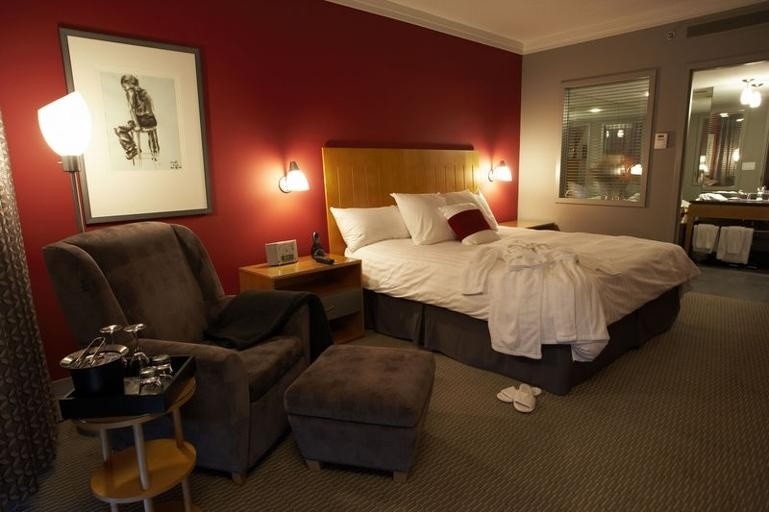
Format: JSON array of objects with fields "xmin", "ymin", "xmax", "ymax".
[
  {"xmin": 99, "ymin": 322, "xmax": 121, "ymax": 345},
  {"xmin": 124, "ymin": 324, "xmax": 149, "ymax": 370}
]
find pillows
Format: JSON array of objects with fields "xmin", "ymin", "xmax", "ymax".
[
  {"xmin": 473, "ymin": 187, "xmax": 500, "ymax": 233},
  {"xmin": 389, "ymin": 188, "xmax": 500, "ymax": 246},
  {"xmin": 329, "ymin": 204, "xmax": 411, "ymax": 254}
]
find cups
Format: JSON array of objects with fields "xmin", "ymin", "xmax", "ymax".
[{"xmin": 130, "ymin": 352, "xmax": 174, "ymax": 394}]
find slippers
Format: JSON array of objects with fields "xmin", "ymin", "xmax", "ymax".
[
  {"xmin": 513, "ymin": 383, "xmax": 536, "ymax": 413},
  {"xmin": 497, "ymin": 386, "xmax": 542, "ymax": 403}
]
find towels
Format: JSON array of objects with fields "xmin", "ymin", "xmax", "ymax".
[
  {"xmin": 691, "ymin": 223, "xmax": 755, "ymax": 265},
  {"xmin": 696, "ymin": 193, "xmax": 728, "ymax": 202}
]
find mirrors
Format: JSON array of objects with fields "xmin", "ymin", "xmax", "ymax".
[{"xmin": 691, "ymin": 109, "xmax": 748, "ymax": 191}]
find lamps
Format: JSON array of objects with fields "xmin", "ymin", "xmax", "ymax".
[
  {"xmin": 740, "ymin": 78, "xmax": 763, "ymax": 108},
  {"xmin": 488, "ymin": 160, "xmax": 512, "ymax": 183},
  {"xmin": 279, "ymin": 161, "xmax": 309, "ymax": 193},
  {"xmin": 37, "ymin": 90, "xmax": 96, "ymax": 235}
]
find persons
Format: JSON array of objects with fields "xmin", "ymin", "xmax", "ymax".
[{"xmin": 114, "ymin": 74, "xmax": 160, "ymax": 161}]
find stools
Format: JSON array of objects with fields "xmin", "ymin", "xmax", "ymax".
[{"xmin": 283, "ymin": 343, "xmax": 436, "ymax": 483}]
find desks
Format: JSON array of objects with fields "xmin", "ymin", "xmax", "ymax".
[{"xmin": 683, "ymin": 198, "xmax": 769, "ymax": 257}]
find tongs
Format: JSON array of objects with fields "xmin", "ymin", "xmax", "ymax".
[{"xmin": 73, "ymin": 336, "xmax": 105, "ymax": 366}]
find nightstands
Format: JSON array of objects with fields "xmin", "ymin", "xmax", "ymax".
[
  {"xmin": 238, "ymin": 253, "xmax": 368, "ymax": 345},
  {"xmin": 498, "ymin": 220, "xmax": 555, "ymax": 230}
]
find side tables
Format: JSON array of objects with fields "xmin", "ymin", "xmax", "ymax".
[{"xmin": 77, "ymin": 376, "xmax": 197, "ymax": 512}]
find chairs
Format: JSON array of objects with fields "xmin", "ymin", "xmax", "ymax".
[{"xmin": 41, "ymin": 220, "xmax": 312, "ymax": 485}]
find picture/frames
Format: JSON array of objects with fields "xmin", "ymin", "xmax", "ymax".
[{"xmin": 58, "ymin": 27, "xmax": 214, "ymax": 226}]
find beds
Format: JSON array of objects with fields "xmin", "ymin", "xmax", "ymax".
[{"xmin": 321, "ymin": 147, "xmax": 702, "ymax": 396}]
